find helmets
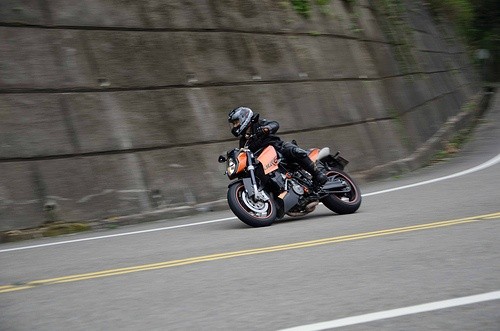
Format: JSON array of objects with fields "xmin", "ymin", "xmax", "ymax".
[{"xmin": 228, "ymin": 106, "xmax": 253, "ymax": 137}]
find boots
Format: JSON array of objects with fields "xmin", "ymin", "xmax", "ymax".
[{"xmin": 299, "ymin": 156, "xmax": 327, "ymax": 185}]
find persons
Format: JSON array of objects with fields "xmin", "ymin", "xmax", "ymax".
[{"xmin": 227, "ymin": 106, "xmax": 329, "ymax": 184}]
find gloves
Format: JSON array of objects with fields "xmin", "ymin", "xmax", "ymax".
[{"xmin": 256, "ymin": 127, "xmax": 269, "ymax": 136}]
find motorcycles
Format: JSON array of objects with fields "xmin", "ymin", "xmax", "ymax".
[{"xmin": 216, "ymin": 145, "xmax": 362, "ymax": 227}]
